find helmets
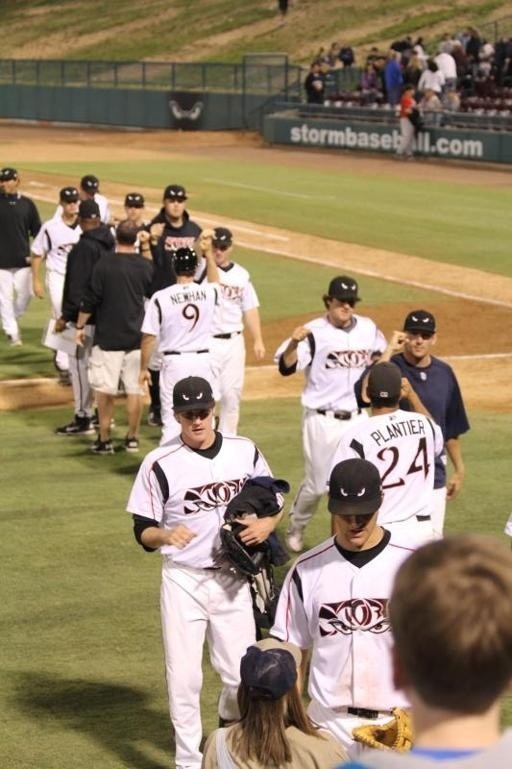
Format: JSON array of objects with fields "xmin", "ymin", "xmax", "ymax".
[{"xmin": 1, "ymin": 167, "xmax": 18, "ymax": 182}]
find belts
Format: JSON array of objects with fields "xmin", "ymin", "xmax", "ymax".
[
  {"xmin": 166, "ymin": 349, "xmax": 208, "ymax": 355},
  {"xmin": 214, "ymin": 333, "xmax": 232, "ymax": 338},
  {"xmin": 318, "ymin": 408, "xmax": 352, "ymax": 420}
]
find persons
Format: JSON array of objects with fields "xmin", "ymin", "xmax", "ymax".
[
  {"xmin": 273, "ymin": 274, "xmax": 391, "ymax": 554},
  {"xmin": 333, "ymin": 533, "xmax": 512, "ymax": 769},
  {"xmin": 32, "ymin": 175, "xmax": 268, "ymax": 458},
  {"xmin": 355, "ymin": 309, "xmax": 471, "ymax": 502},
  {"xmin": 124, "ymin": 375, "xmax": 286, "ymax": 769},
  {"xmin": 268, "ymin": 457, "xmax": 424, "ymax": 755},
  {"xmin": 201, "ymin": 637, "xmax": 354, "ymax": 769},
  {"xmin": 0, "ymin": 167, "xmax": 42, "ymax": 347},
  {"xmin": 300, "ymin": 24, "xmax": 512, "ymax": 161},
  {"xmin": 324, "ymin": 361, "xmax": 446, "ymax": 541}
]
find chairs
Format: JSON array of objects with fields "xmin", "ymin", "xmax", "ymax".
[{"xmin": 321, "ymin": 67, "xmax": 512, "ymax": 127}]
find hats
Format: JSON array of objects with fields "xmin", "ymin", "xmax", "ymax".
[
  {"xmin": 173, "ymin": 377, "xmax": 215, "ymax": 412},
  {"xmin": 368, "ymin": 361, "xmax": 402, "ymax": 400},
  {"xmin": 328, "ymin": 457, "xmax": 382, "ymax": 515},
  {"xmin": 405, "ymin": 309, "xmax": 436, "ymax": 334},
  {"xmin": 59, "ymin": 175, "xmax": 232, "ymax": 267},
  {"xmin": 240, "ymin": 638, "xmax": 302, "ymax": 701},
  {"xmin": 328, "ymin": 274, "xmax": 361, "ymax": 303}
]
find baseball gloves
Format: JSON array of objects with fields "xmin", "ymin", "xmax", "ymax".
[
  {"xmin": 352, "ymin": 707, "xmax": 412, "ymax": 753},
  {"xmin": 219, "ymin": 522, "xmax": 272, "ymax": 575}
]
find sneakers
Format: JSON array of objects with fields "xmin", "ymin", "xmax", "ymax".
[{"xmin": 56, "ymin": 413, "xmax": 140, "ymax": 454}]
